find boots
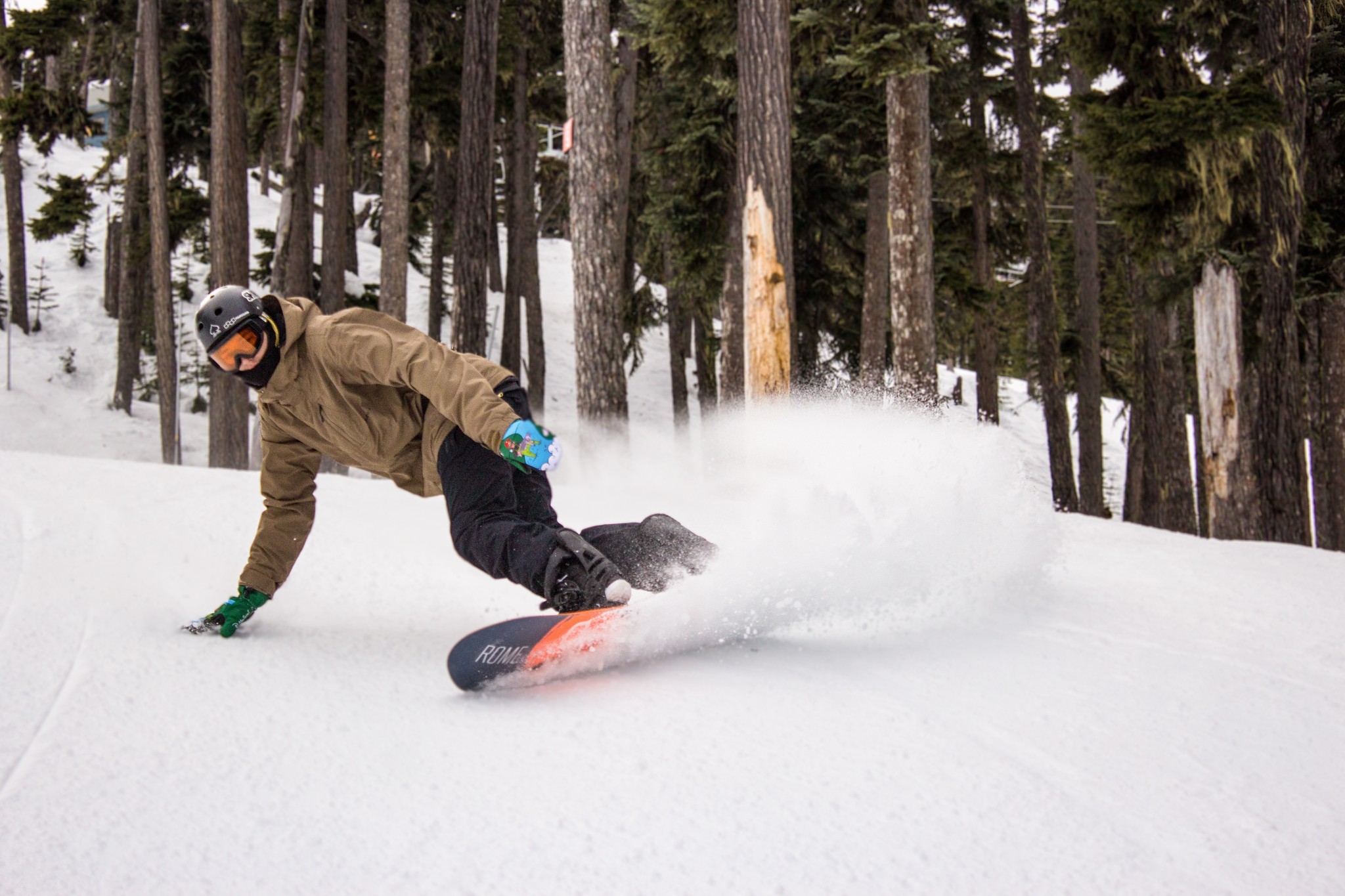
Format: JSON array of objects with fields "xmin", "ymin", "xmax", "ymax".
[
  {"xmin": 540, "ymin": 528, "xmax": 631, "ymax": 616},
  {"xmin": 629, "ymin": 514, "xmax": 723, "ymax": 592}
]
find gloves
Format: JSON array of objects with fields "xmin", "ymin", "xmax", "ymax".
[
  {"xmin": 177, "ymin": 586, "xmax": 271, "ymax": 639},
  {"xmin": 501, "ymin": 419, "xmax": 561, "ymax": 477}
]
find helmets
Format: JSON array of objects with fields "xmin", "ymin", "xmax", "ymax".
[{"xmin": 197, "ymin": 286, "xmax": 264, "ymax": 352}]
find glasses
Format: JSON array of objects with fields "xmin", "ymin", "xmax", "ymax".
[{"xmin": 203, "ymin": 320, "xmax": 264, "ymax": 372}]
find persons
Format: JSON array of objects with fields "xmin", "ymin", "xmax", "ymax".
[{"xmin": 178, "ymin": 284, "xmax": 717, "ymax": 640}]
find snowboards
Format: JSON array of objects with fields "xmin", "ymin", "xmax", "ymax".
[{"xmin": 445, "ymin": 574, "xmax": 797, "ymax": 693}]
